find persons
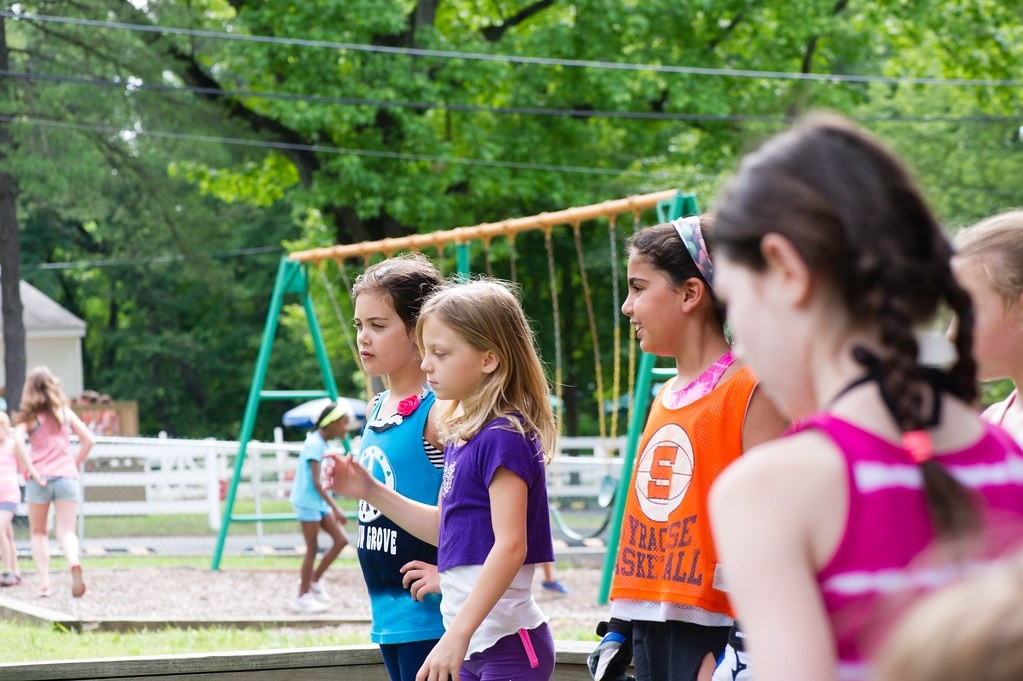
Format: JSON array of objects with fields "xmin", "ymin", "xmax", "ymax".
[
  {"xmin": 351, "ymin": 252, "xmax": 465, "ymax": 681},
  {"xmin": 707, "ymin": 112, "xmax": 1023, "ymax": 681},
  {"xmin": 69, "ymin": 389, "xmax": 119, "ymax": 471},
  {"xmin": 588, "ymin": 214, "xmax": 795, "ymax": 681},
  {"xmin": 869, "ymin": 538, "xmax": 1023, "ymax": 681},
  {"xmin": 289, "ymin": 403, "xmax": 349, "ymax": 615},
  {"xmin": 949, "ymin": 208, "xmax": 1023, "ymax": 453},
  {"xmin": 13, "ymin": 366, "xmax": 95, "ymax": 598},
  {"xmin": 541, "ymin": 563, "xmax": 568, "ymax": 595},
  {"xmin": 322, "ymin": 278, "xmax": 555, "ymax": 681},
  {"xmin": 0, "ymin": 410, "xmax": 46, "ymax": 586}
]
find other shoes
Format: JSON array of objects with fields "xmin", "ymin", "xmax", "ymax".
[
  {"xmin": 1, "ymin": 573, "xmax": 22, "ymax": 586},
  {"xmin": 34, "ymin": 584, "xmax": 47, "ymax": 598},
  {"xmin": 70, "ymin": 562, "xmax": 86, "ymax": 597}
]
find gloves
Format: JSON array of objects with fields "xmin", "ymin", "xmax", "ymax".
[
  {"xmin": 586, "ymin": 618, "xmax": 634, "ymax": 680},
  {"xmin": 710, "ymin": 618, "xmax": 752, "ymax": 681}
]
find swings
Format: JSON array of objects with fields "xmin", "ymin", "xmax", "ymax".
[
  {"xmin": 315, "ymin": 235, "xmax": 520, "ymax": 400},
  {"xmin": 543, "ymin": 219, "xmax": 642, "ymax": 543}
]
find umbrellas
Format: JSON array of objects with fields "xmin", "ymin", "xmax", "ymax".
[{"xmin": 283, "ymin": 397, "xmax": 369, "ymax": 428}]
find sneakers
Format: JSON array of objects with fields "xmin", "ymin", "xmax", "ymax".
[{"xmin": 288, "ymin": 582, "xmax": 328, "ymax": 615}]
